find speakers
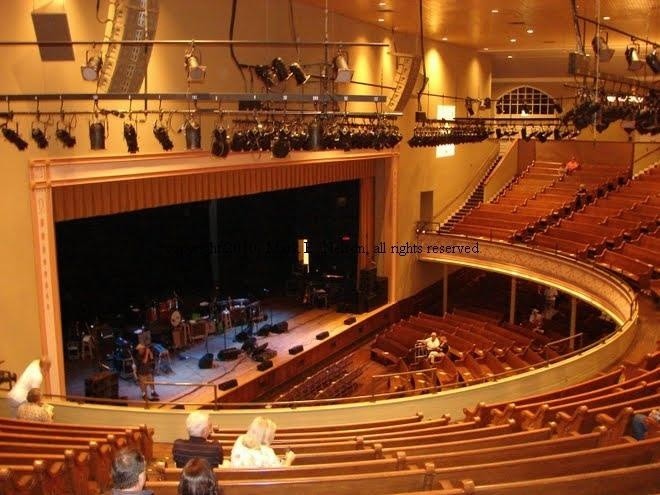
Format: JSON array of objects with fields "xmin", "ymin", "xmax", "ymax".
[
  {"xmin": 344, "ymin": 316, "xmax": 356, "ymax": 325},
  {"xmin": 84, "ymin": 370, "xmax": 119, "ymax": 406},
  {"xmin": 359, "ymin": 266, "xmax": 377, "ymax": 300},
  {"xmin": 289, "ymin": 345, "xmax": 303, "ymax": 355},
  {"xmin": 316, "ymin": 331, "xmax": 329, "ymax": 340},
  {"xmin": 218, "ymin": 379, "xmax": 238, "ymax": 390},
  {"xmin": 198, "ymin": 321, "xmax": 288, "ymax": 372}
]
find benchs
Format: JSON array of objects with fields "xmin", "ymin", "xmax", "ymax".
[
  {"xmin": 453, "ymin": 162, "xmax": 660, "ymax": 299},
  {"xmin": 371, "ymin": 308, "xmax": 578, "ymax": 349}
]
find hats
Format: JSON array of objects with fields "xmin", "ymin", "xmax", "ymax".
[{"xmin": 136, "ymin": 344, "xmax": 145, "ymax": 350}]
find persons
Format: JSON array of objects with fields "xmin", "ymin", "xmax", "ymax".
[
  {"xmin": 6, "ymin": 355, "xmax": 52, "ymax": 416},
  {"xmin": 574, "ymin": 182, "xmax": 587, "ymax": 205},
  {"xmin": 630, "ymin": 408, "xmax": 660, "ymax": 441},
  {"xmin": 229, "ymin": 414, "xmax": 296, "ymax": 469},
  {"xmin": 176, "ymin": 455, "xmax": 221, "ymax": 495},
  {"xmin": 558, "ymin": 162, "xmax": 568, "ymax": 176},
  {"xmin": 416, "ymin": 331, "xmax": 440, "ymax": 351},
  {"xmin": 132, "ymin": 343, "xmax": 160, "ymax": 401},
  {"xmin": 426, "ymin": 336, "xmax": 448, "ymax": 364},
  {"xmin": 16, "ymin": 387, "xmax": 57, "ymax": 424},
  {"xmin": 528, "ymin": 286, "xmax": 559, "ymax": 335},
  {"xmin": 109, "ymin": 446, "xmax": 155, "ymax": 495},
  {"xmin": 172, "ymin": 410, "xmax": 224, "ymax": 470},
  {"xmin": 567, "ymin": 155, "xmax": 579, "ymax": 175}
]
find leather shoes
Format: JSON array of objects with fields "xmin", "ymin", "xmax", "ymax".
[{"xmin": 151, "ymin": 391, "xmax": 159, "ymax": 396}]
